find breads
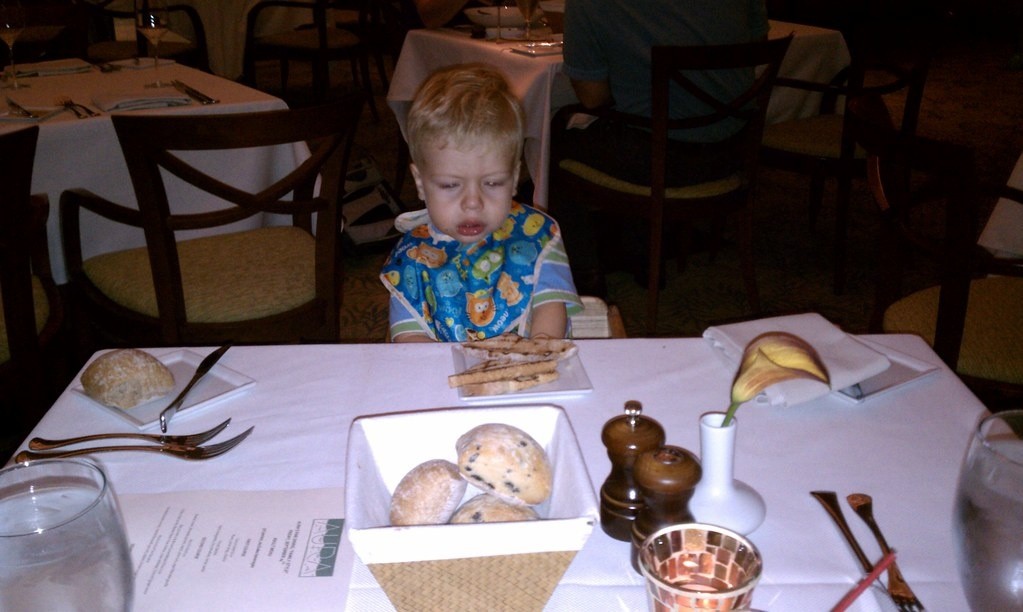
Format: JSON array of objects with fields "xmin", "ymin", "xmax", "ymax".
[
  {"xmin": 445, "ymin": 331, "xmax": 577, "ymax": 398},
  {"xmin": 448, "ymin": 492, "xmax": 540, "ymax": 523},
  {"xmin": 456, "ymin": 422, "xmax": 553, "ymax": 508},
  {"xmin": 388, "ymin": 458, "xmax": 468, "ymax": 525},
  {"xmin": 80, "ymin": 350, "xmax": 175, "ymax": 409}
]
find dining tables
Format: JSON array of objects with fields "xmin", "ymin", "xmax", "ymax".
[
  {"xmin": 385, "ymin": 20, "xmax": 849, "ymax": 215},
  {"xmin": 0, "ymin": 55, "xmax": 322, "ymax": 288},
  {"xmin": 0, "ymin": 334, "xmax": 1023, "ymax": 612}
]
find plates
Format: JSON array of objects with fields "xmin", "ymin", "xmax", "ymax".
[
  {"xmin": 452, "ymin": 338, "xmax": 593, "ymax": 401},
  {"xmin": 0, "ymin": 106, "xmax": 66, "ymax": 121},
  {"xmin": 108, "ymin": 57, "xmax": 176, "ymax": 70},
  {"xmin": 69, "ymin": 348, "xmax": 257, "ymax": 431},
  {"xmin": 510, "ymin": 45, "xmax": 563, "ymax": 57},
  {"xmin": 831, "ymin": 332, "xmax": 944, "ymax": 404}
]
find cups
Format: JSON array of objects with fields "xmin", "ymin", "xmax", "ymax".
[
  {"xmin": 953, "ymin": 409, "xmax": 1022, "ymax": 612},
  {"xmin": 637, "ymin": 525, "xmax": 762, "ymax": 612},
  {"xmin": 1, "ymin": 455, "xmax": 132, "ymax": 611}
]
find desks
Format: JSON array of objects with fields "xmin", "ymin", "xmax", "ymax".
[{"xmin": 164, "ymin": 0, "xmax": 369, "ymax": 81}]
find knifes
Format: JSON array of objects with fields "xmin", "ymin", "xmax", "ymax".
[
  {"xmin": 172, "ymin": 78, "xmax": 218, "ymax": 105},
  {"xmin": 809, "ymin": 491, "xmax": 897, "ymax": 612},
  {"xmin": 159, "ymin": 342, "xmax": 233, "ymax": 434}
]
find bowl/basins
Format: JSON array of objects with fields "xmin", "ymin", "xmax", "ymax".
[
  {"xmin": 464, "ymin": 6, "xmax": 543, "ymax": 28},
  {"xmin": 539, "ymin": 2, "xmax": 564, "ymax": 34}
]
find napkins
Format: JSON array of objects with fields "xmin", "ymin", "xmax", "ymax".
[
  {"xmin": 90, "ymin": 87, "xmax": 191, "ymax": 111},
  {"xmin": 36, "ymin": 58, "xmax": 92, "ymax": 76},
  {"xmin": 702, "ymin": 312, "xmax": 890, "ymax": 407}
]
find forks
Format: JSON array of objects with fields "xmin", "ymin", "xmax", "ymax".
[
  {"xmin": 53, "ymin": 96, "xmax": 100, "ymax": 119},
  {"xmin": 14, "ymin": 425, "xmax": 255, "ymax": 465},
  {"xmin": 847, "ymin": 494, "xmax": 926, "ymax": 612},
  {"xmin": 29, "ymin": 418, "xmax": 232, "ymax": 451}
]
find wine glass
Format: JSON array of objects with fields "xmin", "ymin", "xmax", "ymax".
[
  {"xmin": 486, "ymin": 0, "xmax": 508, "ymax": 43},
  {"xmin": 134, "ymin": 0, "xmax": 174, "ymax": 89},
  {"xmin": 514, "ymin": 0, "xmax": 539, "ymax": 43},
  {"xmin": 0, "ymin": 0, "xmax": 29, "ymax": 90}
]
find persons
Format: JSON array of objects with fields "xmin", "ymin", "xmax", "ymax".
[
  {"xmin": 374, "ymin": 0, "xmax": 493, "ymax": 70},
  {"xmin": 540, "ymin": 0, "xmax": 769, "ymax": 265},
  {"xmin": 378, "ymin": 64, "xmax": 585, "ymax": 343}
]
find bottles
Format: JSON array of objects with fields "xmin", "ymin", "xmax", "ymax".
[
  {"xmin": 600, "ymin": 400, "xmax": 665, "ymax": 542},
  {"xmin": 632, "ymin": 447, "xmax": 703, "ymax": 575}
]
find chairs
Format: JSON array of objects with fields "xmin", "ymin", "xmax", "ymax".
[
  {"xmin": 547, "ymin": 30, "xmax": 796, "ymax": 339},
  {"xmin": 78, "ymin": 1, "xmax": 215, "ymax": 75},
  {"xmin": 740, "ymin": 0, "xmax": 954, "ymax": 296},
  {"xmin": 0, "ymin": 124, "xmax": 64, "ymax": 447},
  {"xmin": 241, "ymin": 0, "xmax": 391, "ymax": 123},
  {"xmin": 868, "ymin": 181, "xmax": 1022, "ymax": 415},
  {"xmin": 57, "ymin": 93, "xmax": 368, "ymax": 376}
]
